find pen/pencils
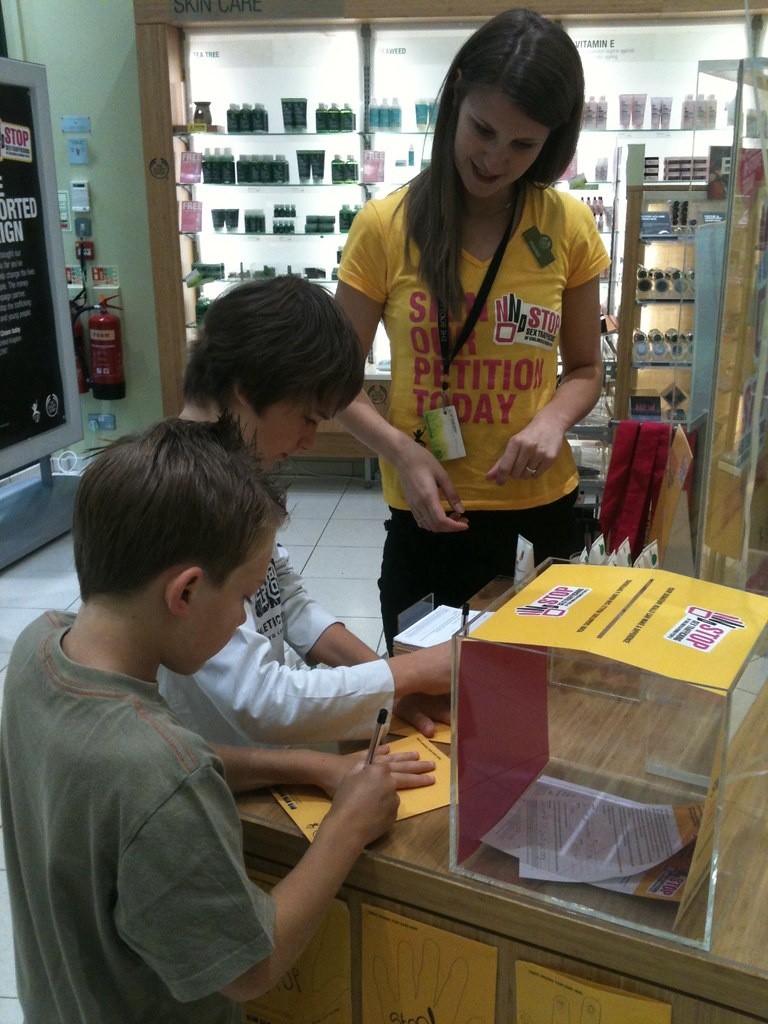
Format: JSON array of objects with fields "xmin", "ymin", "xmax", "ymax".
[
  {"xmin": 361, "ymin": 708, "xmax": 388, "ymax": 771},
  {"xmin": 462, "ymin": 601, "xmax": 471, "ymax": 638}
]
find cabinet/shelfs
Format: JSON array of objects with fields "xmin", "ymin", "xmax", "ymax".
[
  {"xmin": 131, "ymin": 0, "xmax": 767, "ymax": 600},
  {"xmin": 235, "ymin": 575, "xmax": 767, "ymax": 1023}
]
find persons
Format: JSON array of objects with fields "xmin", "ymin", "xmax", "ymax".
[
  {"xmin": 0, "ymin": 275, "xmax": 453, "ymax": 1024},
  {"xmin": 331, "ymin": 9, "xmax": 606, "ymax": 657}
]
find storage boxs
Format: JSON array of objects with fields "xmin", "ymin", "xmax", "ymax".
[{"xmin": 449, "ymin": 555, "xmax": 767, "ymax": 953}]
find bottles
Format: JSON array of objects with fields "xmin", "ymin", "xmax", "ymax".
[
  {"xmin": 339, "ymin": 205, "xmax": 362, "ymax": 233},
  {"xmin": 331, "ymin": 155, "xmax": 358, "ymax": 185},
  {"xmin": 369, "ymin": 98, "xmax": 401, "ymax": 132},
  {"xmin": 631, "ymin": 328, "xmax": 693, "ymax": 361},
  {"xmin": 195, "ymin": 296, "xmax": 209, "ymax": 325},
  {"xmin": 272, "ymin": 204, "xmax": 296, "ymax": 234},
  {"xmin": 305, "ymin": 215, "xmax": 335, "ymax": 233},
  {"xmin": 227, "ymin": 104, "xmax": 268, "ymax": 133},
  {"xmin": 582, "ymin": 95, "xmax": 607, "ymax": 131},
  {"xmin": 316, "ymin": 103, "xmax": 357, "ymax": 132},
  {"xmin": 638, "ymin": 267, "xmax": 695, "ymax": 295},
  {"xmin": 682, "ymin": 94, "xmax": 717, "ymax": 130},
  {"xmin": 580, "ymin": 197, "xmax": 604, "ymax": 232},
  {"xmin": 202, "ymin": 147, "xmax": 289, "ymax": 184},
  {"xmin": 244, "ymin": 209, "xmax": 266, "ymax": 234}
]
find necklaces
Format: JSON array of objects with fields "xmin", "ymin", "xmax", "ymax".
[{"xmin": 464, "ymin": 194, "xmax": 517, "ymax": 219}]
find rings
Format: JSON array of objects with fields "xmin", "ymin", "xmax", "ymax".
[{"xmin": 526, "ymin": 466, "xmax": 537, "ymax": 474}]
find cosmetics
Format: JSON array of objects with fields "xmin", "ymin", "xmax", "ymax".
[
  {"xmin": 181, "ymin": 89, "xmax": 739, "ymax": 423},
  {"xmin": 511, "ymin": 535, "xmax": 662, "ymax": 595}
]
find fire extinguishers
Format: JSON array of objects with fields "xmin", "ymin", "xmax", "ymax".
[{"xmin": 70, "ymin": 289, "xmax": 126, "ymax": 399}]
